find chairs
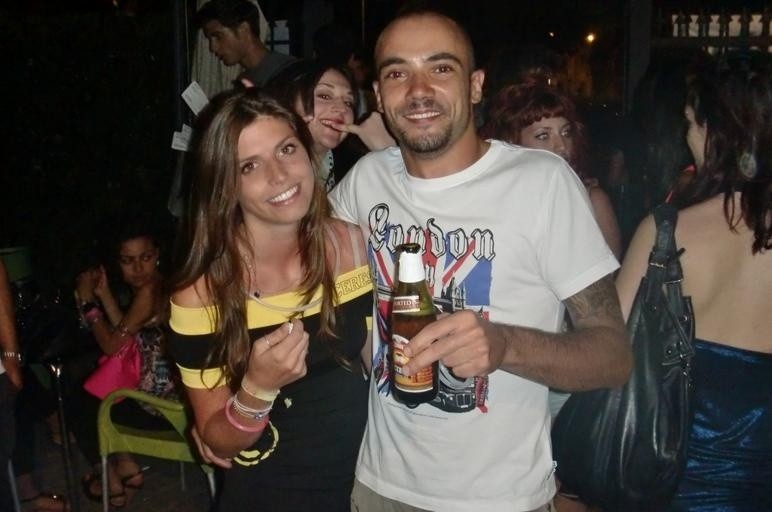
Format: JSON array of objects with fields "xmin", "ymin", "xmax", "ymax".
[
  {"xmin": 0, "ymin": 246, "xmax": 39, "ymax": 285},
  {"xmin": 97, "ymin": 388, "xmax": 218, "ymax": 511}
]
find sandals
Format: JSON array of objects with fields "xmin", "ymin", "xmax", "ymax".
[
  {"xmin": 85, "ymin": 465, "xmax": 142, "ymax": 508},
  {"xmin": 21, "ymin": 493, "xmax": 75, "ymax": 511}
]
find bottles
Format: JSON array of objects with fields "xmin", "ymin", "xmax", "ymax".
[{"xmin": 385, "ymin": 242, "xmax": 440, "ymax": 404}]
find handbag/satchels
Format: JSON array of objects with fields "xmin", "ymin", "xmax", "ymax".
[
  {"xmin": 549, "ymin": 275, "xmax": 695, "ymax": 510},
  {"xmin": 83, "ymin": 337, "xmax": 143, "ymax": 404}
]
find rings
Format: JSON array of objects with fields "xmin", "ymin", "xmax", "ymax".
[{"xmin": 264, "ymin": 334, "xmax": 274, "ymax": 347}]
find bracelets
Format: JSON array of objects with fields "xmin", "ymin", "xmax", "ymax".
[
  {"xmin": 225, "ymin": 397, "xmax": 269, "ymax": 432},
  {"xmin": 233, "ymin": 391, "xmax": 274, "ymax": 420},
  {"xmin": 241, "ymin": 374, "xmax": 280, "ymax": 401}
]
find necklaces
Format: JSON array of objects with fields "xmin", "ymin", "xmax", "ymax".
[
  {"xmin": 241, "ymin": 244, "xmax": 307, "ymax": 299},
  {"xmin": 241, "ymin": 218, "xmax": 341, "ymax": 311}
]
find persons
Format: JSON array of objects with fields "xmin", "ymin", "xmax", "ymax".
[
  {"xmin": 2, "ymin": 223, "xmax": 175, "ymax": 510},
  {"xmin": 197, "ymin": 1, "xmax": 400, "ymax": 194},
  {"xmin": 191, "ymin": 2, "xmax": 634, "ymax": 511},
  {"xmin": 478, "ymin": 50, "xmax": 696, "ymax": 282},
  {"xmin": 160, "ymin": 88, "xmax": 374, "ymax": 510},
  {"xmin": 615, "ymin": 49, "xmax": 770, "ymax": 510}
]
